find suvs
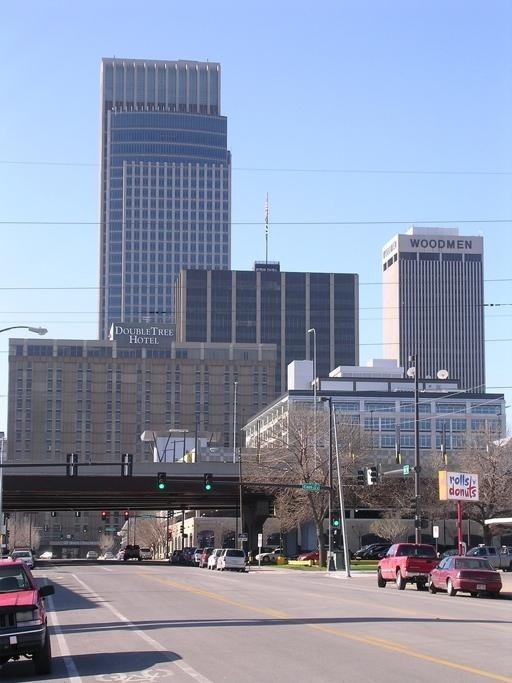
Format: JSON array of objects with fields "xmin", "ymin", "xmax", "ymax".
[{"xmin": 1, "ymin": 560, "xmax": 57, "ymax": 676}]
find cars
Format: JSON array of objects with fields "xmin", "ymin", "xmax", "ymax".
[
  {"xmin": 103, "ymin": 544, "xmax": 153, "ymax": 561},
  {"xmin": 427, "ymin": 556, "xmax": 502, "ymax": 598},
  {"xmin": 87, "ymin": 550, "xmax": 99, "ymax": 559},
  {"xmin": 298, "ymin": 544, "xmax": 352, "ymax": 561},
  {"xmin": 169, "ymin": 545, "xmax": 247, "ymax": 572},
  {"xmin": 255, "ymin": 548, "xmax": 286, "ymax": 563},
  {"xmin": 40, "ymin": 551, "xmax": 57, "ymax": 559},
  {"xmin": 354, "ymin": 542, "xmax": 391, "ymax": 559},
  {"xmin": 12, "ymin": 547, "xmax": 35, "ymax": 570}
]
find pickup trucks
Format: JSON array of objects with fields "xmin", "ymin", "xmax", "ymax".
[
  {"xmin": 376, "ymin": 543, "xmax": 440, "ymax": 591},
  {"xmin": 464, "ymin": 545, "xmax": 512, "ymax": 572}
]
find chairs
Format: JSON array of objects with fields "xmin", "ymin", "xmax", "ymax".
[{"xmin": 1, "ymin": 576, "xmax": 19, "ymax": 590}]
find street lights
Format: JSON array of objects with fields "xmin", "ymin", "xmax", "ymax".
[
  {"xmin": 407, "ymin": 353, "xmax": 420, "ymax": 542},
  {"xmin": 306, "ymin": 327, "xmax": 319, "ymax": 474}
]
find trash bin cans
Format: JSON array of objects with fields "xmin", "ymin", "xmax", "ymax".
[{"xmin": 333, "ymin": 551, "xmax": 345, "ymax": 570}]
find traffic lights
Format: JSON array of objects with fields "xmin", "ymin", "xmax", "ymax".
[
  {"xmin": 124, "ymin": 510, "xmax": 129, "ymax": 520},
  {"xmin": 203, "ymin": 471, "xmax": 214, "ymax": 492},
  {"xmin": 157, "ymin": 471, "xmax": 168, "ymax": 490},
  {"xmin": 101, "ymin": 511, "xmax": 107, "ymax": 520},
  {"xmin": 409, "ymin": 496, "xmax": 418, "ymax": 515},
  {"xmin": 356, "ymin": 468, "xmax": 364, "ymax": 485},
  {"xmin": 370, "ymin": 466, "xmax": 378, "ymax": 482},
  {"xmin": 332, "ymin": 518, "xmax": 340, "ymax": 528}
]
